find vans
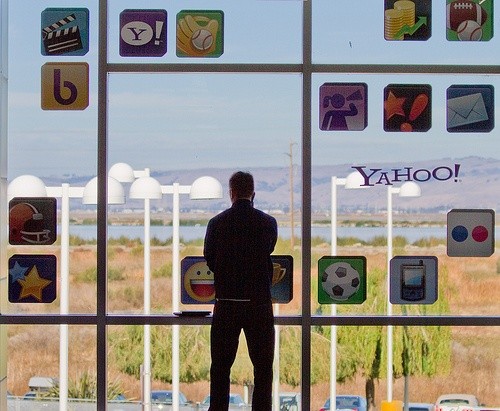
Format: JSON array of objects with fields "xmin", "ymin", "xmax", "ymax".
[{"xmin": 22, "ymin": 375, "xmax": 57, "ymax": 401}]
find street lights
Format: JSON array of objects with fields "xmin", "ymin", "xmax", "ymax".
[{"xmin": 283, "ymin": 139, "xmax": 297, "ymax": 248}]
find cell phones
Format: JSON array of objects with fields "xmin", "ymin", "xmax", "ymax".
[
  {"xmin": 250, "ymin": 192, "xmax": 255, "ymax": 208},
  {"xmin": 400, "ymin": 260, "xmax": 426, "ymax": 302}
]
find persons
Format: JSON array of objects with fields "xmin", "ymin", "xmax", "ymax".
[{"xmin": 203, "ymin": 171, "xmax": 277, "ymax": 411}]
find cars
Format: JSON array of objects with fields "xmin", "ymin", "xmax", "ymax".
[
  {"xmin": 279, "ymin": 392, "xmax": 301, "ymax": 411},
  {"xmin": 319, "ymin": 395, "xmax": 368, "ymax": 411},
  {"xmin": 151, "ymin": 390, "xmax": 193, "ymax": 406},
  {"xmin": 202, "ymin": 394, "xmax": 245, "ymax": 406},
  {"xmin": 433, "ymin": 393, "xmax": 479, "ymax": 411}
]
